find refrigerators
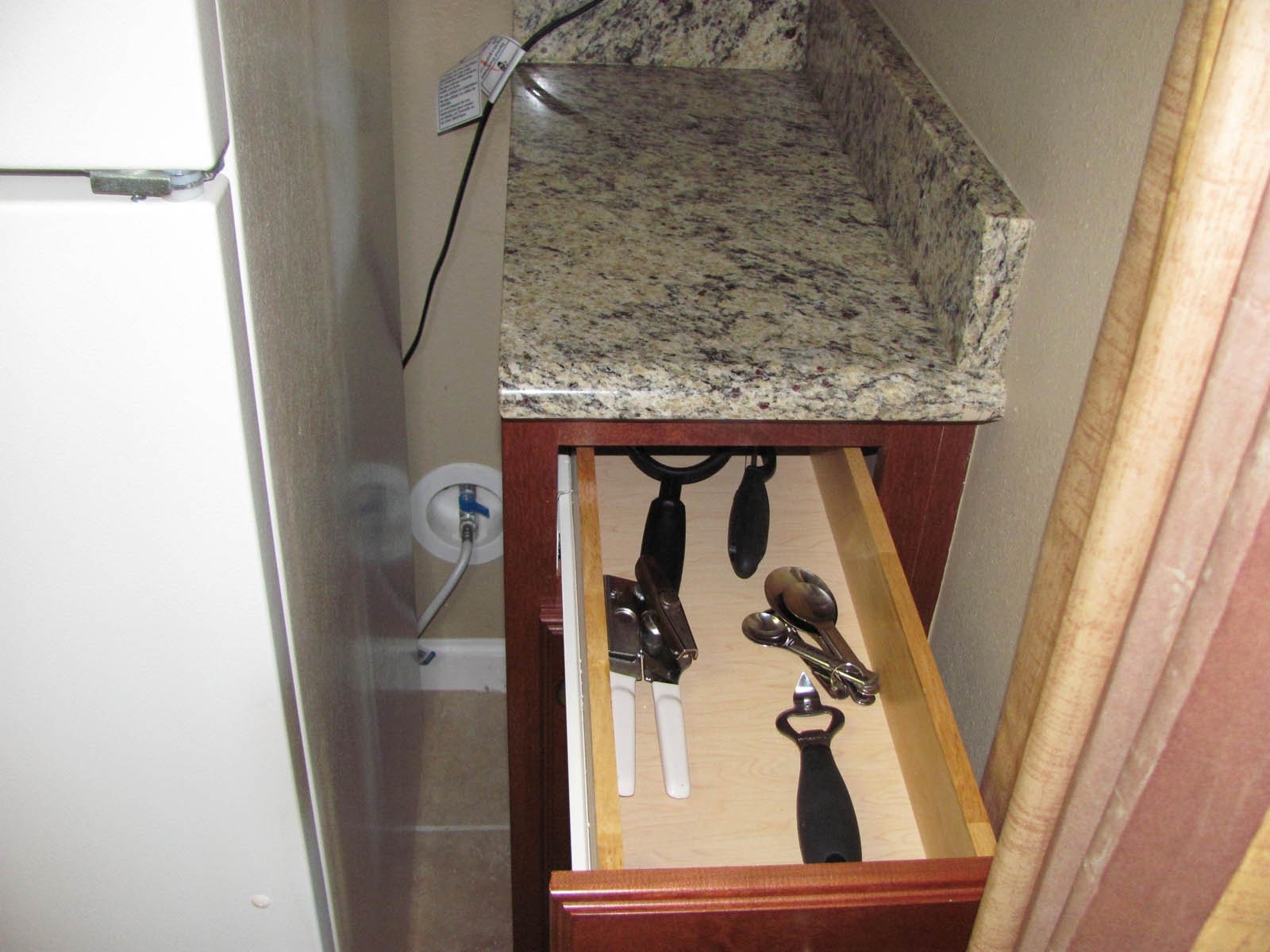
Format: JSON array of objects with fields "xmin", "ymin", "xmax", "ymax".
[{"xmin": 0, "ymin": 0, "xmax": 367, "ymax": 952}]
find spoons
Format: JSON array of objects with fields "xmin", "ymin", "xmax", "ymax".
[{"xmin": 742, "ymin": 566, "xmax": 881, "ymax": 705}]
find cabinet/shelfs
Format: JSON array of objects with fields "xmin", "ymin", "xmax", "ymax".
[{"xmin": 490, "ymin": 2, "xmax": 1028, "ymax": 952}]
